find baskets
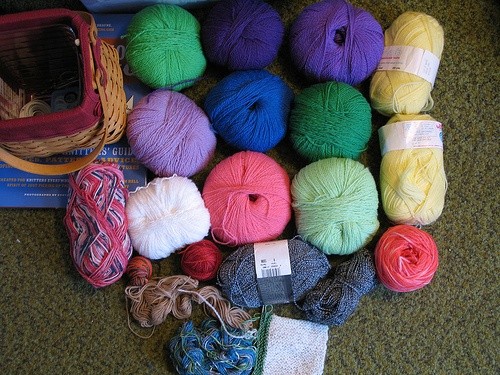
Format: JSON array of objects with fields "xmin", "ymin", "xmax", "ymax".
[{"xmin": 0, "ymin": 11, "xmax": 128, "ymax": 178}]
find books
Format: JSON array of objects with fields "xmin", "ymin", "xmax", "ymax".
[{"xmin": 0, "ymin": 12, "xmax": 150, "ymax": 209}]
